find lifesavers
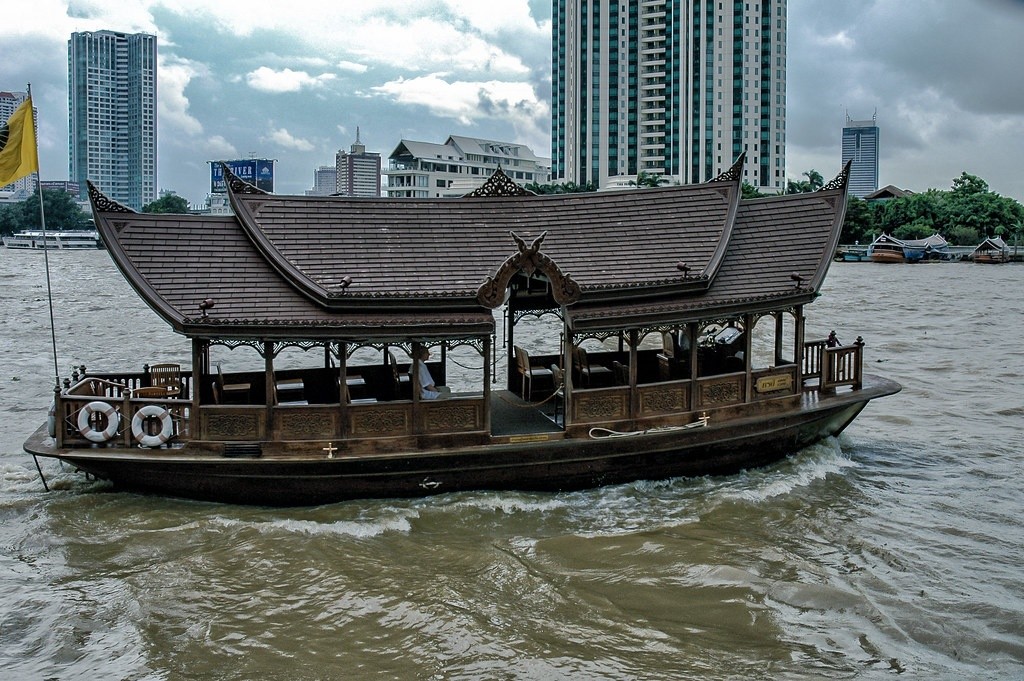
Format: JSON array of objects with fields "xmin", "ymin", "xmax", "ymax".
[
  {"xmin": 78, "ymin": 401, "xmax": 119, "ymax": 441},
  {"xmin": 131, "ymin": 405, "xmax": 173, "ymax": 447}
]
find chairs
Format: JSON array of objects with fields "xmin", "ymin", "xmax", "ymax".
[
  {"xmin": 151, "ymin": 363, "xmax": 182, "ymax": 399},
  {"xmin": 551, "ymin": 364, "xmax": 564, "ymax": 424},
  {"xmin": 273, "ymin": 371, "xmax": 305, "ymax": 401},
  {"xmin": 331, "ymin": 358, "xmax": 378, "ymax": 402},
  {"xmin": 576, "ymin": 347, "xmax": 629, "ymax": 388},
  {"xmin": 217, "ymin": 361, "xmax": 250, "ymax": 398},
  {"xmin": 389, "ymin": 352, "xmax": 422, "ymax": 400},
  {"xmin": 273, "ymin": 385, "xmax": 308, "ymax": 405},
  {"xmin": 133, "ymin": 386, "xmax": 167, "ymax": 436},
  {"xmin": 513, "ymin": 345, "xmax": 554, "ymax": 402},
  {"xmin": 212, "ymin": 382, "xmax": 220, "ymax": 405}
]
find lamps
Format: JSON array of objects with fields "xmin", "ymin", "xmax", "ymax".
[
  {"xmin": 198, "ymin": 299, "xmax": 214, "ymax": 317},
  {"xmin": 339, "ymin": 276, "xmax": 353, "ymax": 294},
  {"xmin": 791, "ymin": 271, "xmax": 805, "ymax": 289},
  {"xmin": 677, "ymin": 261, "xmax": 691, "ymax": 278}
]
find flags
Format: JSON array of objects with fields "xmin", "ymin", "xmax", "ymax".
[{"xmin": 0, "ymin": 99, "xmax": 39, "ymax": 188}]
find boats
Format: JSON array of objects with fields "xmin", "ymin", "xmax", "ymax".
[
  {"xmin": 967, "ymin": 235, "xmax": 1013, "ymax": 264},
  {"xmin": 21, "ymin": 144, "xmax": 905, "ymax": 506},
  {"xmin": 1, "ymin": 229, "xmax": 106, "ymax": 250},
  {"xmin": 834, "ymin": 231, "xmax": 963, "ymax": 264}
]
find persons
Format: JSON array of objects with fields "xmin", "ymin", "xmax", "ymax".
[
  {"xmin": 409, "ymin": 346, "xmax": 451, "ymax": 400},
  {"xmin": 680, "ymin": 324, "xmax": 717, "ymax": 352}
]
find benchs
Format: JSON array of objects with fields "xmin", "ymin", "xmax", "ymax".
[{"xmin": 65, "ymin": 377, "xmax": 126, "ymax": 436}]
